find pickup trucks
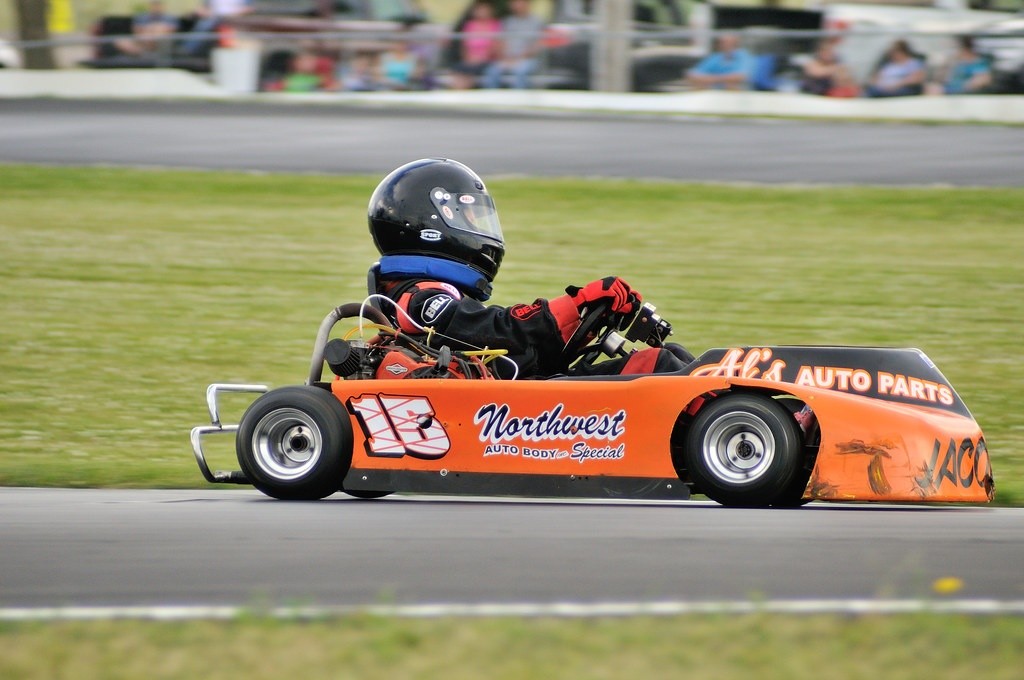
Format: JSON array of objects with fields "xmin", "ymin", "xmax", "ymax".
[
  {"xmin": 693, "ymin": 0, "xmax": 1024, "ymax": 92},
  {"xmin": 78, "ymin": 0, "xmax": 426, "ymax": 95}
]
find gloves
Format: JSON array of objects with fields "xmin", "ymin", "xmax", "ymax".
[{"xmin": 574, "ymin": 276, "xmax": 641, "ymax": 330}]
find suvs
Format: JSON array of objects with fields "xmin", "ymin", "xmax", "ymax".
[{"xmin": 421, "ymin": 0, "xmax": 823, "ymax": 88}]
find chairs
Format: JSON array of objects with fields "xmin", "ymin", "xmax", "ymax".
[{"xmin": 367, "ymin": 261, "xmax": 396, "ymax": 309}]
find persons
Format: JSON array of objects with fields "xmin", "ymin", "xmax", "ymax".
[
  {"xmin": 130, "ymin": 0, "xmax": 994, "ymax": 98},
  {"xmin": 367, "ymin": 157, "xmax": 695, "ymax": 380}
]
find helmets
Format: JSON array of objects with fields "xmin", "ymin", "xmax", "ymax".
[{"xmin": 368, "ymin": 158, "xmax": 505, "ymax": 301}]
